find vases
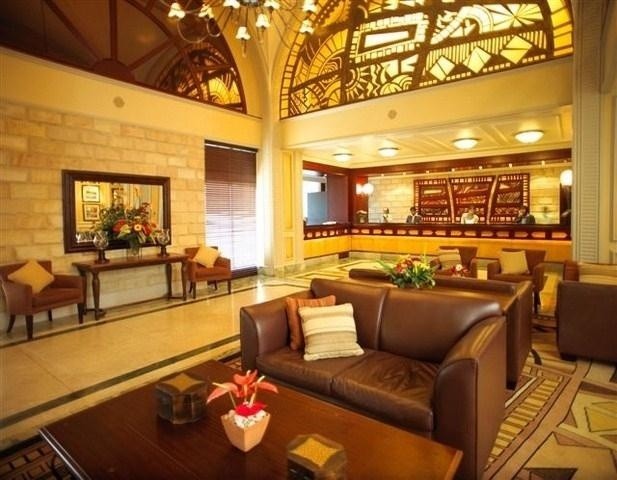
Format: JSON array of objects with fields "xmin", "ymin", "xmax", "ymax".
[
  {"xmin": 221, "ymin": 411, "xmax": 270, "ymax": 451},
  {"xmin": 127, "ymin": 239, "xmax": 142, "ymax": 260}
]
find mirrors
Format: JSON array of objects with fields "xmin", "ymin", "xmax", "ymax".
[{"xmin": 61, "ymin": 169, "xmax": 171, "ymax": 254}]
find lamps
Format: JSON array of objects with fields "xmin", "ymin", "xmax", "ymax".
[
  {"xmin": 159, "ymin": 0, "xmax": 317, "ymax": 58},
  {"xmin": 334, "ymin": 153, "xmax": 352, "ymax": 162},
  {"xmin": 379, "ymin": 148, "xmax": 398, "ymax": 156},
  {"xmin": 515, "ymin": 131, "xmax": 544, "ymax": 143},
  {"xmin": 453, "ymin": 137, "xmax": 479, "ymax": 149}
]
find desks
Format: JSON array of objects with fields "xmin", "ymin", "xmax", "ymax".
[{"xmin": 71, "ymin": 254, "xmax": 190, "ymax": 320}]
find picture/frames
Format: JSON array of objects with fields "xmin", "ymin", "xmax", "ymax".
[
  {"xmin": 111, "ymin": 188, "xmax": 124, "ymax": 205},
  {"xmin": 82, "ymin": 185, "xmax": 101, "ymax": 202},
  {"xmin": 83, "ymin": 203, "xmax": 100, "ymax": 221}
]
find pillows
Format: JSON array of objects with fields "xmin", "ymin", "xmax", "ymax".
[
  {"xmin": 8, "ymin": 261, "xmax": 55, "ymax": 293},
  {"xmin": 285, "ymin": 296, "xmax": 340, "ymax": 350},
  {"xmin": 495, "ymin": 251, "xmax": 530, "ymax": 274},
  {"xmin": 436, "ymin": 248, "xmax": 461, "ymax": 271},
  {"xmin": 192, "ymin": 245, "xmax": 221, "ymax": 268},
  {"xmin": 298, "ymin": 303, "xmax": 364, "ymax": 361}
]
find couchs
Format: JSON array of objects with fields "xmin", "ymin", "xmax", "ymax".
[
  {"xmin": 349, "ymin": 269, "xmax": 533, "ymax": 391},
  {"xmin": 557, "ymin": 260, "xmax": 617, "ymax": 364},
  {"xmin": 240, "ymin": 278, "xmax": 506, "ymax": 479},
  {"xmin": 0, "ymin": 260, "xmax": 83, "ymax": 340},
  {"xmin": 184, "ymin": 246, "xmax": 232, "ymax": 299},
  {"xmin": 430, "ymin": 246, "xmax": 478, "ymax": 279},
  {"xmin": 487, "ymin": 249, "xmax": 546, "ymax": 290}
]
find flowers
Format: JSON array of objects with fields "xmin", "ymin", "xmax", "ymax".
[
  {"xmin": 207, "ymin": 369, "xmax": 280, "ymax": 416},
  {"xmin": 97, "ymin": 205, "xmax": 126, "ymax": 238},
  {"xmin": 112, "ymin": 202, "xmax": 157, "ymax": 240}
]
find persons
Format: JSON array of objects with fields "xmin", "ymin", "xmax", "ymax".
[
  {"xmin": 517, "ymin": 207, "xmax": 535, "ymax": 224},
  {"xmin": 381, "ymin": 207, "xmax": 391, "ymax": 221},
  {"xmin": 406, "ymin": 208, "xmax": 421, "ymax": 223},
  {"xmin": 461, "ymin": 205, "xmax": 478, "ymax": 223}
]
